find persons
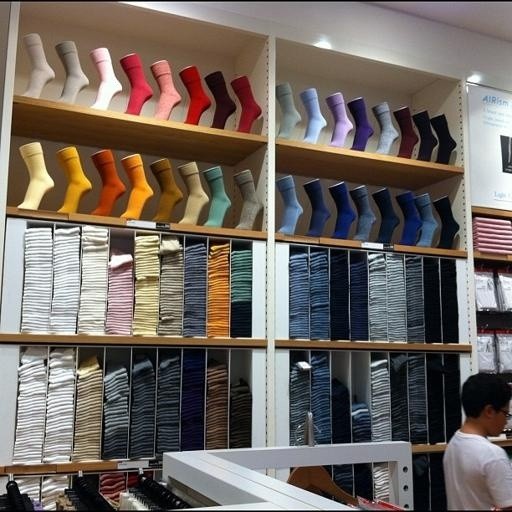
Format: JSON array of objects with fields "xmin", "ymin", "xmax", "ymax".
[{"xmin": 443, "ymin": 373, "xmax": 512, "ymax": 512}]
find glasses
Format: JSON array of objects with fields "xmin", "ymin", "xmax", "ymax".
[{"xmin": 493, "ymin": 408, "xmax": 512, "ymax": 420}]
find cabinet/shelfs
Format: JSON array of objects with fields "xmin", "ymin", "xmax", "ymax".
[
  {"xmin": 270, "ymin": 37, "xmax": 472, "ymax": 512},
  {"xmin": 469, "ymin": 205, "xmax": 512, "ymax": 445},
  {"xmin": 0, "ymin": 1, "xmax": 270, "ymax": 511}
]
